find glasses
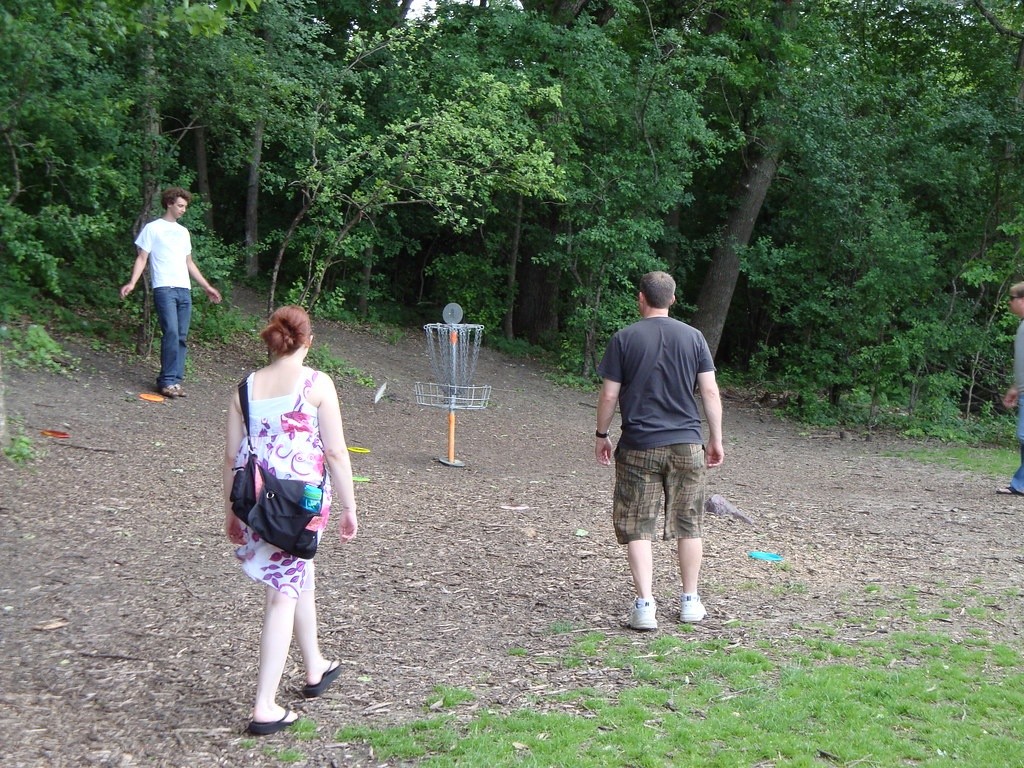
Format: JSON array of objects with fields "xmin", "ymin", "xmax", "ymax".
[{"xmin": 1010, "ymin": 295, "xmax": 1022, "ymax": 301}]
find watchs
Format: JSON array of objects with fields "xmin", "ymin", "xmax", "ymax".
[{"xmin": 595, "ymin": 428, "xmax": 610, "ymax": 437}]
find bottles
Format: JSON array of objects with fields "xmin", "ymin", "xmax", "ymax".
[{"xmin": 299, "ymin": 482, "xmax": 321, "ymax": 514}]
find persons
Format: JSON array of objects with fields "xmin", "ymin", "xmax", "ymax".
[
  {"xmin": 223, "ymin": 306, "xmax": 358, "ymax": 734},
  {"xmin": 997, "ymin": 282, "xmax": 1024, "ymax": 495},
  {"xmin": 121, "ymin": 187, "xmax": 222, "ymax": 398},
  {"xmin": 595, "ymin": 271, "xmax": 723, "ymax": 636}
]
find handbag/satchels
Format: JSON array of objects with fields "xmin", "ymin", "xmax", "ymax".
[{"xmin": 230, "ymin": 454, "xmax": 323, "ymax": 559}]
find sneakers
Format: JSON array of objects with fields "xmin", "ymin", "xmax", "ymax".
[
  {"xmin": 175, "ymin": 383, "xmax": 189, "ymax": 397},
  {"xmin": 630, "ymin": 600, "xmax": 658, "ymax": 628},
  {"xmin": 160, "ymin": 385, "xmax": 179, "ymax": 397},
  {"xmin": 680, "ymin": 596, "xmax": 706, "ymax": 624}
]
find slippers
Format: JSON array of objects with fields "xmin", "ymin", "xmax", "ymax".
[
  {"xmin": 997, "ymin": 487, "xmax": 1020, "ymax": 495},
  {"xmin": 249, "ymin": 707, "xmax": 300, "ymax": 734},
  {"xmin": 301, "ymin": 659, "xmax": 342, "ymax": 698}
]
